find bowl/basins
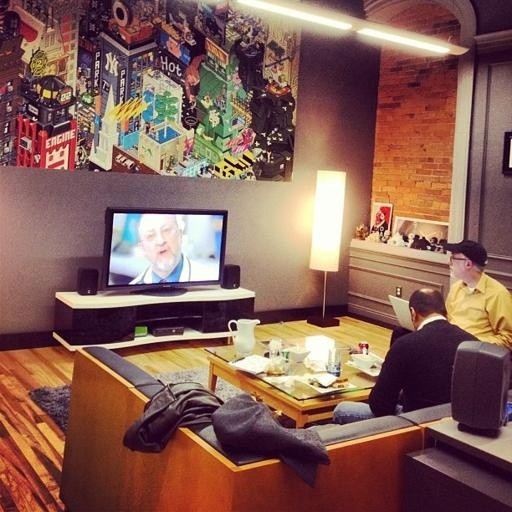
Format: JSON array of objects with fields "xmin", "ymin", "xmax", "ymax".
[
  {"xmin": 350, "ymin": 353, "xmax": 379, "ymax": 370},
  {"xmin": 281, "ymin": 347, "xmax": 312, "ymax": 363}
]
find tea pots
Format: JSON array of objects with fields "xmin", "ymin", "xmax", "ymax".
[{"xmin": 228, "ymin": 318, "xmax": 261, "ymax": 355}]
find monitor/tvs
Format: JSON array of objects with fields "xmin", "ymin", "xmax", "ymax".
[{"xmin": 102, "ymin": 207, "xmax": 228, "ymax": 296}]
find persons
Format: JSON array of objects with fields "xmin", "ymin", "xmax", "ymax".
[
  {"xmin": 401, "ymin": 230, "xmax": 448, "ymax": 254},
  {"xmin": 332, "ymin": 287, "xmax": 483, "ymax": 425},
  {"xmin": 389, "ymin": 239, "xmax": 511, "ymax": 350},
  {"xmin": 129, "ymin": 213, "xmax": 219, "ymax": 285}
]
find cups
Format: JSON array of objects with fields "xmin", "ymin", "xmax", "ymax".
[{"xmin": 327, "ymin": 348, "xmax": 341, "ymax": 378}]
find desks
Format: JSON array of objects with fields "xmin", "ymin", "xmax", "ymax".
[{"xmin": 407, "ymin": 418, "xmax": 512, "ymax": 511}]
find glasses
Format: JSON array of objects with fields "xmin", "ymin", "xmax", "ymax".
[{"xmin": 450, "ymin": 255, "xmax": 467, "ymax": 262}]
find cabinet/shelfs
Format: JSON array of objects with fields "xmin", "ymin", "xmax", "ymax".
[{"xmin": 53, "ymin": 284, "xmax": 255, "ymax": 352}]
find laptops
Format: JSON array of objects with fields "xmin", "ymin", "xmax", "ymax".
[{"xmin": 388, "ymin": 295, "xmax": 416, "ymax": 332}]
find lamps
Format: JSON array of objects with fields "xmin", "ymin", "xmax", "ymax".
[
  {"xmin": 307, "ymin": 170, "xmax": 348, "ymax": 327},
  {"xmin": 236, "ymin": 0, "xmax": 470, "ymax": 57}
]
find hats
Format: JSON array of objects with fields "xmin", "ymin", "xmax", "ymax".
[{"xmin": 447, "ymin": 240, "xmax": 488, "ymax": 267}]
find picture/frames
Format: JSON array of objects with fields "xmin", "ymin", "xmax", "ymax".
[
  {"xmin": 370, "ymin": 202, "xmax": 393, "ymax": 236},
  {"xmin": 502, "ymin": 131, "xmax": 512, "ymax": 175}
]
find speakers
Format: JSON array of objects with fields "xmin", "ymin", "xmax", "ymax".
[
  {"xmin": 77, "ymin": 268, "xmax": 98, "ymax": 295},
  {"xmin": 221, "ymin": 264, "xmax": 240, "ymax": 289},
  {"xmin": 450, "ymin": 341, "xmax": 511, "ymax": 439}
]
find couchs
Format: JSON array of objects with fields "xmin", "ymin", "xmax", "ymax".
[{"xmin": 59, "ymin": 346, "xmax": 512, "ymax": 512}]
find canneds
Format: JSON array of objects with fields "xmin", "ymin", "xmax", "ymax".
[{"xmin": 359, "ymin": 342, "xmax": 368, "ymax": 355}]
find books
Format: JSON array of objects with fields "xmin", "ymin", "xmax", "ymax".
[{"xmin": 227, "ymin": 353, "xmax": 270, "ymax": 375}]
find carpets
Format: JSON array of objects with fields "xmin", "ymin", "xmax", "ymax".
[{"xmin": 30, "ymin": 368, "xmax": 242, "ymax": 435}]
